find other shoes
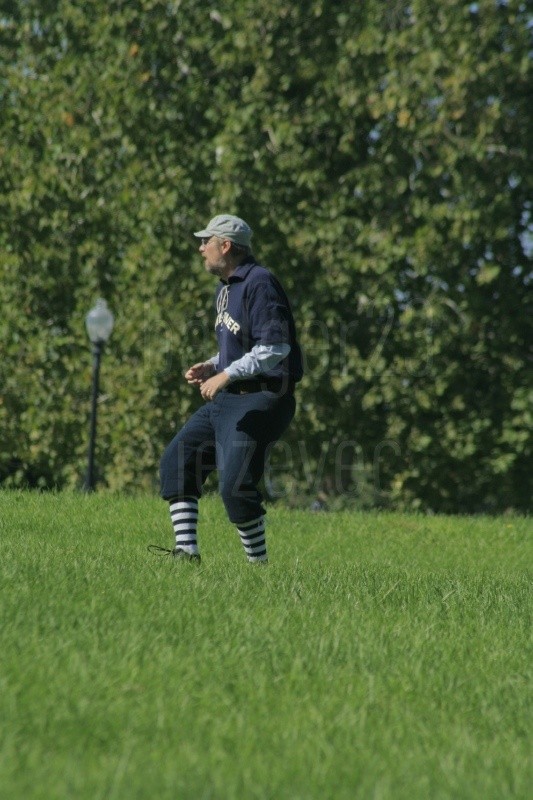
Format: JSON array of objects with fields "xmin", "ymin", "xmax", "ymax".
[{"xmin": 147, "ymin": 544, "xmax": 200, "ymax": 566}]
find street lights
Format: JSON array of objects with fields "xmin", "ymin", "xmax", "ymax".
[{"xmin": 85, "ymin": 297, "xmax": 115, "ymax": 495}]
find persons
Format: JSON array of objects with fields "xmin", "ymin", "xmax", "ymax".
[{"xmin": 159, "ymin": 214, "xmax": 304, "ymax": 567}]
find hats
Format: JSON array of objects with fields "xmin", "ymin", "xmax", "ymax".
[{"xmin": 194, "ymin": 214, "xmax": 251, "ymax": 248}]
